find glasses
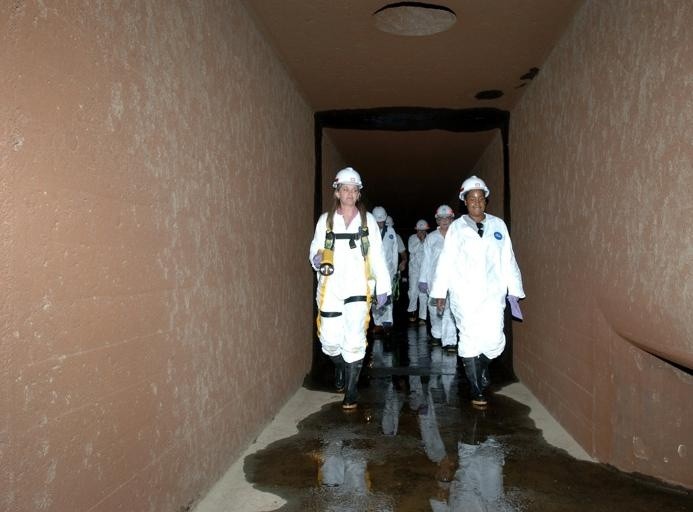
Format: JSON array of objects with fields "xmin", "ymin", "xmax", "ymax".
[{"xmin": 477, "ymin": 222, "xmax": 484, "ymax": 237}]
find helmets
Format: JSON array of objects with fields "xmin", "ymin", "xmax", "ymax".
[
  {"xmin": 372, "ymin": 207, "xmax": 387, "ymax": 222},
  {"xmin": 333, "ymin": 168, "xmax": 363, "ymax": 189},
  {"xmin": 435, "ymin": 205, "xmax": 455, "ymax": 218},
  {"xmin": 414, "ymin": 219, "xmax": 430, "ymax": 230},
  {"xmin": 458, "ymin": 176, "xmax": 490, "ymax": 201},
  {"xmin": 385, "ymin": 216, "xmax": 394, "ymax": 226}
]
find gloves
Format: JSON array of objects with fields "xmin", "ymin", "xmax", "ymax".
[
  {"xmin": 312, "ymin": 249, "xmax": 323, "ymax": 268},
  {"xmin": 418, "ymin": 283, "xmax": 427, "ymax": 292},
  {"xmin": 509, "ymin": 297, "xmax": 525, "ymax": 320},
  {"xmin": 377, "ymin": 294, "xmax": 387, "ymax": 310}
]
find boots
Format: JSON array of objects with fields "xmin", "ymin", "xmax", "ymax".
[
  {"xmin": 328, "ymin": 354, "xmax": 345, "ymax": 392},
  {"xmin": 460, "ymin": 354, "xmax": 493, "ymax": 405},
  {"xmin": 341, "ymin": 359, "xmax": 363, "ymax": 409}
]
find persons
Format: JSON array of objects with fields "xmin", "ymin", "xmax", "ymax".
[
  {"xmin": 367, "ymin": 205, "xmax": 397, "ymax": 334},
  {"xmin": 430, "ymin": 175, "xmax": 526, "ymax": 405},
  {"xmin": 406, "ymin": 217, "xmax": 429, "ymax": 322},
  {"xmin": 308, "ymin": 165, "xmax": 392, "ymax": 408},
  {"xmin": 384, "ymin": 214, "xmax": 407, "ymax": 300},
  {"xmin": 418, "ymin": 204, "xmax": 459, "ymax": 354}
]
